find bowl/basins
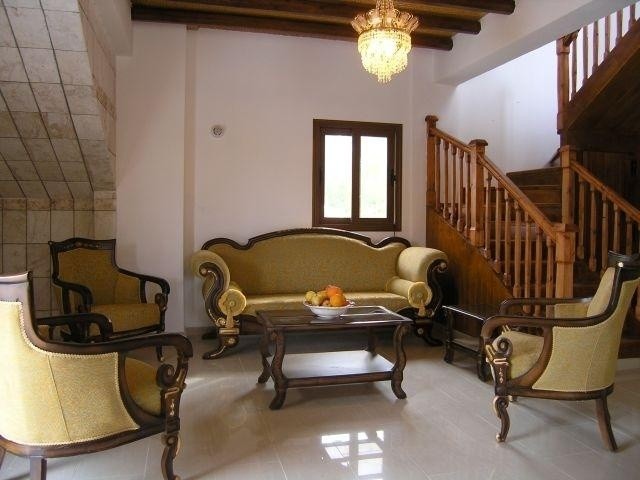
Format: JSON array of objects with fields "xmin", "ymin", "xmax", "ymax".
[{"xmin": 302, "ymin": 300, "xmax": 353, "ymax": 320}]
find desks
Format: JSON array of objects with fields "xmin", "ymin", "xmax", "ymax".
[{"xmin": 437, "ymin": 305, "xmax": 517, "ymax": 383}]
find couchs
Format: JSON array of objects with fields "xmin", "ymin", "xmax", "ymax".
[{"xmin": 182, "ymin": 224, "xmax": 448, "ymax": 360}]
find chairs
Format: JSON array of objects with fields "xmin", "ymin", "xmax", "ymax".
[
  {"xmin": 45, "ymin": 236, "xmax": 171, "ymax": 366},
  {"xmin": 1, "ymin": 268, "xmax": 195, "ymax": 478},
  {"xmin": 474, "ymin": 249, "xmax": 640, "ymax": 453}
]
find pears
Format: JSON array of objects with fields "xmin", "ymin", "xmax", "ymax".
[{"xmin": 306, "ymin": 289, "xmax": 328, "ymax": 306}]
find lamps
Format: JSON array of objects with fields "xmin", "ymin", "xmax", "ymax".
[{"xmin": 348, "ymin": 0, "xmax": 419, "ymax": 83}]
